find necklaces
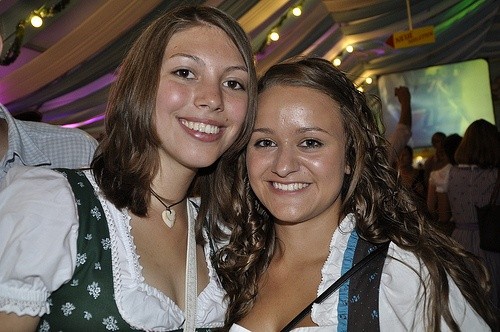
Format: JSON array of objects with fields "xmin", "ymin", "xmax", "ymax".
[{"xmin": 146, "ymin": 184, "xmax": 189, "ymax": 227}]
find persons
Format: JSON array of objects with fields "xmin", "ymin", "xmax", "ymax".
[
  {"xmin": 0, "ymin": 6, "xmax": 257, "ymax": 332},
  {"xmin": 220, "ymin": 58, "xmax": 499, "ymax": 332},
  {"xmin": 425, "ymin": 132, "xmax": 446, "ymax": 172},
  {"xmin": 428, "ymin": 134, "xmax": 457, "ymax": 230},
  {"xmin": 447, "ymin": 119, "xmax": 499, "ymax": 287},
  {"xmin": 0, "ymin": 103, "xmax": 100, "ymax": 190},
  {"xmin": 397, "ymin": 149, "xmax": 426, "ymax": 220},
  {"xmin": 382, "ymin": 86, "xmax": 412, "ymax": 159}
]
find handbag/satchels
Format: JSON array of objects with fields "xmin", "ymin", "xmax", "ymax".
[{"xmin": 475, "ymin": 174, "xmax": 500, "ymax": 254}]
